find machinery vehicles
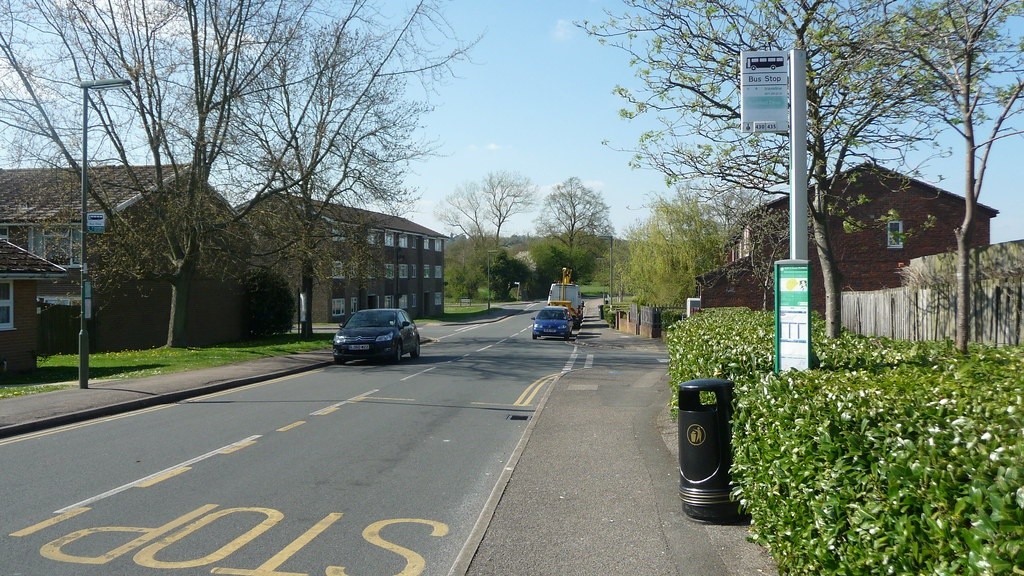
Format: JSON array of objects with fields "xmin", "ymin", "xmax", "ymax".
[{"xmin": 546, "ymin": 267, "xmax": 585, "ymax": 329}]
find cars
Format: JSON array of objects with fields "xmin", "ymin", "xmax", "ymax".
[
  {"xmin": 333, "ymin": 307, "xmax": 421, "ymax": 364},
  {"xmin": 531, "ymin": 306, "xmax": 576, "ymax": 342}
]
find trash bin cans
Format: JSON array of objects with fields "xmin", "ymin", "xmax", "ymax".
[
  {"xmin": 678, "ymin": 380, "xmax": 741, "ymax": 524},
  {"xmin": 598, "ymin": 305, "xmax": 604, "ymax": 319}
]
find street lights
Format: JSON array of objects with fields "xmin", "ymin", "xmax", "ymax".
[
  {"xmin": 487, "ymin": 250, "xmax": 499, "ymax": 311},
  {"xmin": 598, "ymin": 235, "xmax": 614, "ymax": 307},
  {"xmin": 76, "ymin": 77, "xmax": 131, "ymax": 389}
]
